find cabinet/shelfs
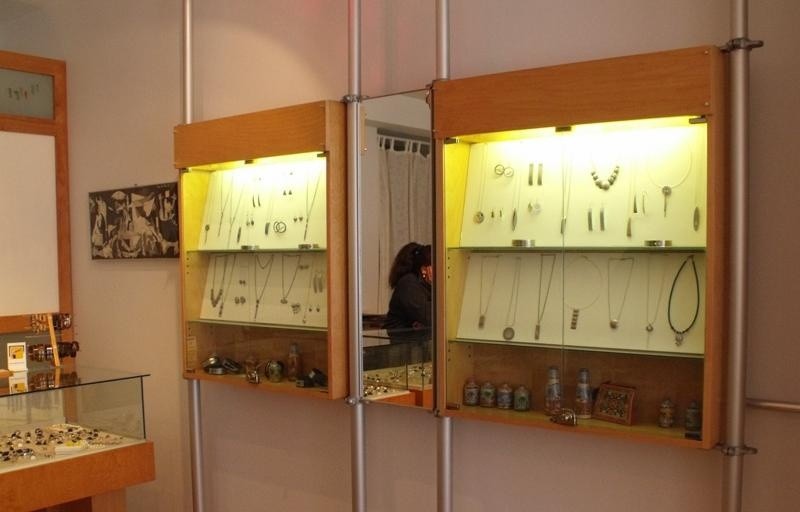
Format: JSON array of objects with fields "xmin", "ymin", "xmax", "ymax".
[
  {"xmin": 173, "ymin": 100, "xmax": 349, "ymax": 400},
  {"xmin": 0, "ymin": 364, "xmax": 156, "ymax": 512},
  {"xmin": 362, "ymin": 327, "xmax": 432, "ymax": 409},
  {"xmin": 432, "ymin": 44, "xmax": 730, "ymax": 451},
  {"xmin": 361, "ymin": 335, "xmax": 416, "ymax": 406}
]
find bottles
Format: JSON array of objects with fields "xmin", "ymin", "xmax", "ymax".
[
  {"xmin": 265, "ymin": 360, "xmax": 285, "ymax": 382},
  {"xmin": 574, "ymin": 369, "xmax": 593, "ymax": 421},
  {"xmin": 686, "ymin": 399, "xmax": 700, "ymax": 431},
  {"xmin": 658, "ymin": 398, "xmax": 677, "ymax": 428},
  {"xmin": 463, "ymin": 381, "xmax": 532, "ymax": 414},
  {"xmin": 286, "ymin": 343, "xmax": 302, "ymax": 381},
  {"xmin": 543, "ymin": 364, "xmax": 562, "ymax": 417}
]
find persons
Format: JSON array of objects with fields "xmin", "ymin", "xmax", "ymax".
[{"xmin": 385, "ymin": 241, "xmax": 432, "ymax": 364}]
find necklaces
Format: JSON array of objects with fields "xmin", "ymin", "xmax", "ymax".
[{"xmin": 204, "ymin": 172, "xmax": 322, "ymax": 324}]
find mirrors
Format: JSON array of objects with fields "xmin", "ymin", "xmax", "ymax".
[{"xmin": 359, "ymin": 88, "xmax": 436, "ymax": 411}]
